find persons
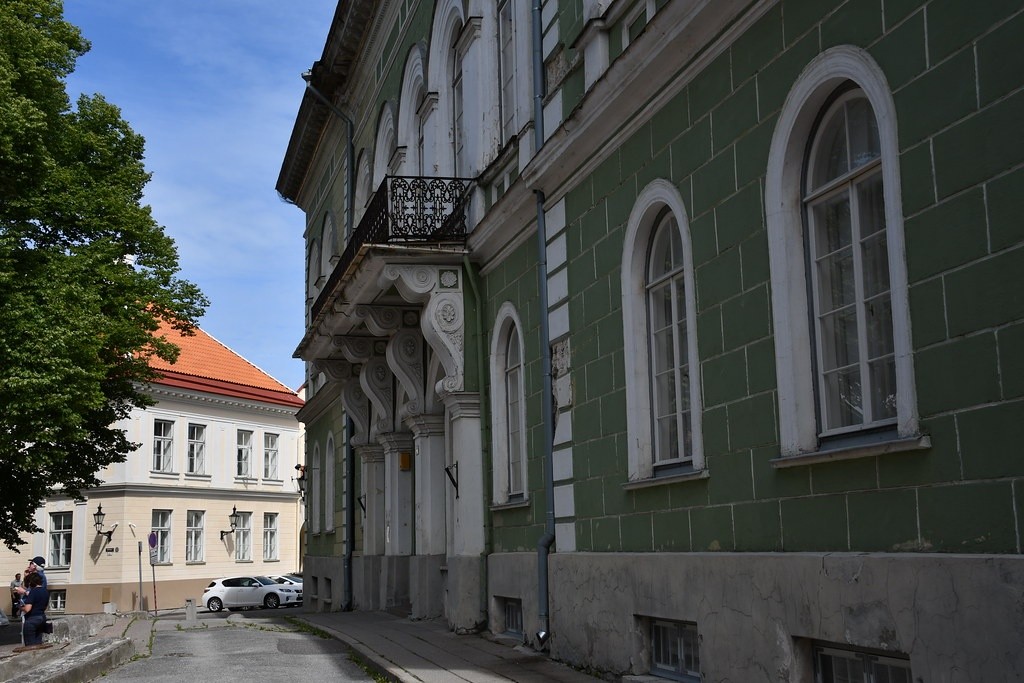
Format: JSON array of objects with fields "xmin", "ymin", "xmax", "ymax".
[{"xmin": 10, "ymin": 557, "xmax": 49, "ymax": 647}]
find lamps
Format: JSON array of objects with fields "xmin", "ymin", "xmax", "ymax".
[
  {"xmin": 93, "ymin": 502, "xmax": 112, "ymax": 541},
  {"xmin": 297, "ymin": 477, "xmax": 306, "ymax": 494},
  {"xmin": 220, "ymin": 504, "xmax": 240, "ymax": 540}
]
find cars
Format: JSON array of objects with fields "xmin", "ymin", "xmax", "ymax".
[
  {"xmin": 201, "ymin": 575, "xmax": 303, "ymax": 612},
  {"xmin": 267, "ymin": 576, "xmax": 304, "ymax": 607}
]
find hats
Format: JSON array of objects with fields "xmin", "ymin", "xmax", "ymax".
[{"xmin": 28, "ymin": 557, "xmax": 45, "ymax": 568}]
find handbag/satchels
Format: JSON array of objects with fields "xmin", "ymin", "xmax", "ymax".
[{"xmin": 43, "ymin": 623, "xmax": 53, "ymax": 634}]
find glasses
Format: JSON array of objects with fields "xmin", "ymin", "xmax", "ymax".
[{"xmin": 30, "ymin": 562, "xmax": 33, "ymax": 565}]
